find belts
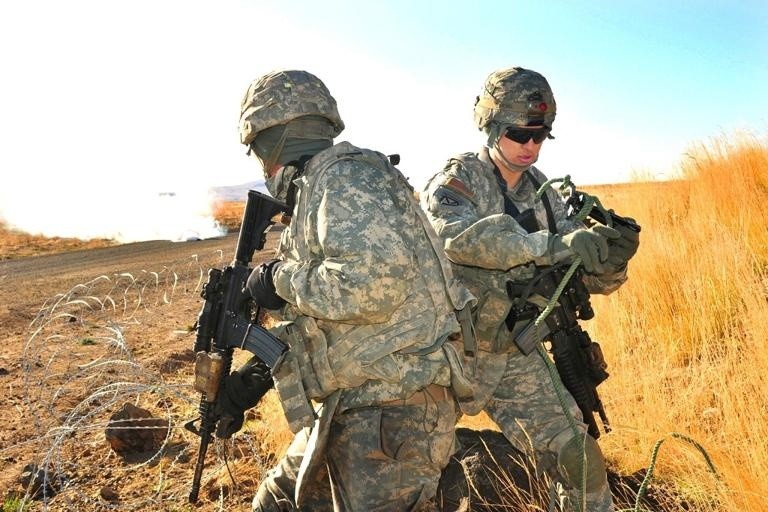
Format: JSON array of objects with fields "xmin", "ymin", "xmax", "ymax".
[{"xmin": 380, "ymin": 383, "xmax": 454, "ymax": 408}]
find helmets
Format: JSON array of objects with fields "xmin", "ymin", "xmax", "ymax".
[
  {"xmin": 474, "ymin": 67, "xmax": 557, "ymax": 130},
  {"xmin": 240, "ymin": 69, "xmax": 345, "ymax": 143}
]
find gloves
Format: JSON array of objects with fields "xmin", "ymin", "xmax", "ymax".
[
  {"xmin": 548, "ymin": 222, "xmax": 619, "ymax": 275},
  {"xmin": 245, "ymin": 261, "xmax": 286, "ymax": 310},
  {"xmin": 218, "ymin": 363, "xmax": 272, "ymax": 437},
  {"xmin": 608, "ymin": 223, "xmax": 639, "ymax": 266}
]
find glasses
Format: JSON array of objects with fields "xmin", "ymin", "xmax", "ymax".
[{"xmin": 502, "ymin": 125, "xmax": 550, "ymax": 143}]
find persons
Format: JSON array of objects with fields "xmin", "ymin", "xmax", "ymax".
[
  {"xmin": 421, "ymin": 67, "xmax": 640, "ymax": 512},
  {"xmin": 207, "ymin": 68, "xmax": 506, "ymax": 512}
]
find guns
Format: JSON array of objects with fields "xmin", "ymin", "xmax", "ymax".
[
  {"xmin": 506, "ymin": 209, "xmax": 612, "ymax": 438},
  {"xmin": 190, "ymin": 190, "xmax": 292, "ymax": 503}
]
